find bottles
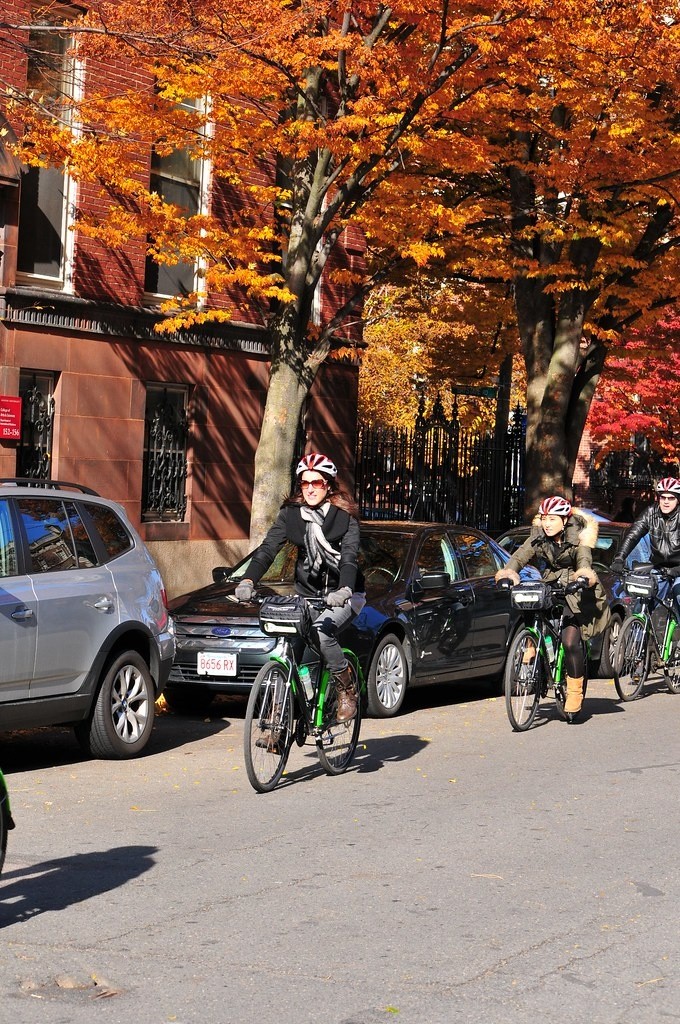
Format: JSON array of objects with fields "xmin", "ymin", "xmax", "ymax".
[
  {"xmin": 632, "ymin": 596, "xmax": 643, "ymax": 614},
  {"xmin": 545, "ymin": 635, "xmax": 554, "ymax": 663},
  {"xmin": 624, "ymin": 598, "xmax": 633, "ymax": 619},
  {"xmin": 297, "ymin": 665, "xmax": 314, "ymax": 701}
]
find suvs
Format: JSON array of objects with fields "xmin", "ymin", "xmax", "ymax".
[{"xmin": 0, "ymin": 476, "xmax": 175, "ymax": 762}]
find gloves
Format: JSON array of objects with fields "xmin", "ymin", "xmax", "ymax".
[
  {"xmin": 496, "ymin": 577, "xmax": 514, "ymax": 595},
  {"xmin": 610, "ymin": 559, "xmax": 624, "ymax": 574},
  {"xmin": 661, "ymin": 567, "xmax": 680, "ymax": 579},
  {"xmin": 234, "ymin": 581, "xmax": 257, "ymax": 601},
  {"xmin": 324, "ymin": 587, "xmax": 352, "ymax": 607},
  {"xmin": 565, "ymin": 580, "xmax": 587, "ymax": 595}
]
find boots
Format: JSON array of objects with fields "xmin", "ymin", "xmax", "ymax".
[
  {"xmin": 332, "ymin": 668, "xmax": 358, "ymax": 723},
  {"xmin": 518, "ymin": 638, "xmax": 535, "ymax": 664},
  {"xmin": 255, "ymin": 704, "xmax": 283, "ymax": 749},
  {"xmin": 564, "ymin": 676, "xmax": 583, "ymax": 712}
]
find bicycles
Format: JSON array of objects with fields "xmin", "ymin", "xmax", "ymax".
[
  {"xmin": 496, "ymin": 573, "xmax": 597, "ymax": 730},
  {"xmin": 604, "ymin": 563, "xmax": 680, "ymax": 703},
  {"xmin": 222, "ymin": 586, "xmax": 369, "ymax": 793}
]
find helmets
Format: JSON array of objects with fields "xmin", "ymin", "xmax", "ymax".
[
  {"xmin": 656, "ymin": 477, "xmax": 680, "ymax": 493},
  {"xmin": 296, "ymin": 454, "xmax": 337, "ymax": 477},
  {"xmin": 538, "ymin": 497, "xmax": 573, "ymax": 516}
]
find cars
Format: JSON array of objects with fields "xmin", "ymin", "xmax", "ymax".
[
  {"xmin": 161, "ymin": 520, "xmax": 540, "ymax": 719},
  {"xmin": 469, "ymin": 522, "xmax": 648, "ymax": 679}
]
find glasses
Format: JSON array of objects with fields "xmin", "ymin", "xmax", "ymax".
[
  {"xmin": 297, "ymin": 479, "xmax": 330, "ymax": 489},
  {"xmin": 660, "ymin": 495, "xmax": 676, "ymax": 502}
]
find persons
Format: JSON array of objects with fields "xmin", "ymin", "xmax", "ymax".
[
  {"xmin": 497, "ymin": 496, "xmax": 613, "ymax": 713},
  {"xmin": 610, "ymin": 476, "xmax": 680, "ymax": 684},
  {"xmin": 236, "ymin": 453, "xmax": 365, "ymax": 750}
]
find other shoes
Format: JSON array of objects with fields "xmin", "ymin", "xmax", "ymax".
[{"xmin": 632, "ymin": 667, "xmax": 645, "ymax": 680}]
